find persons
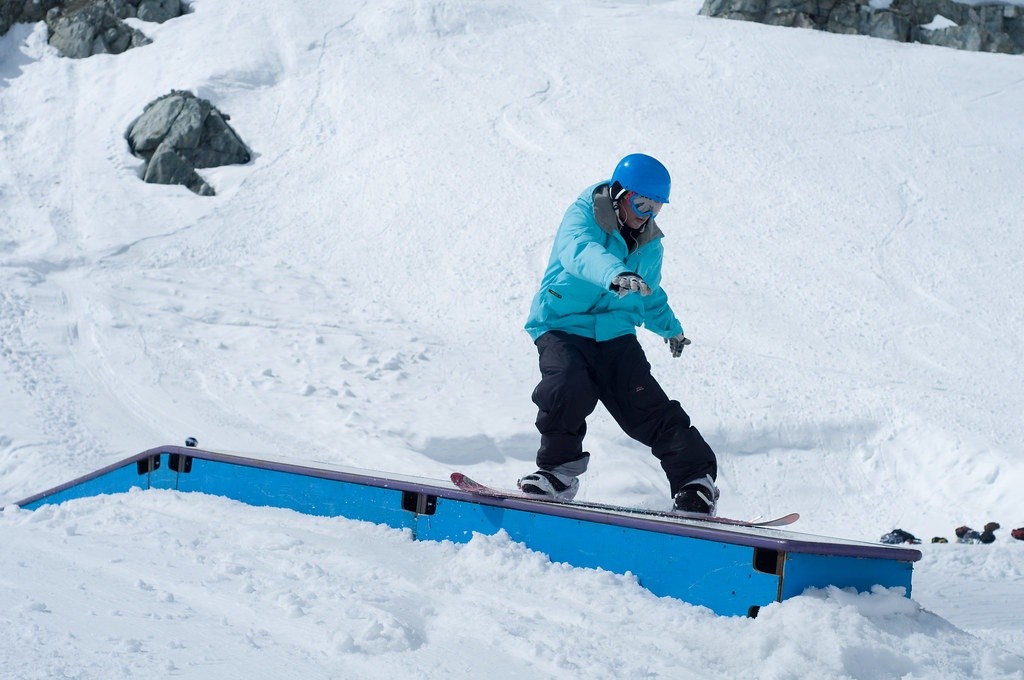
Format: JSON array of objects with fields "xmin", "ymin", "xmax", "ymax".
[{"xmin": 518, "ymin": 153, "xmax": 718, "ymax": 515}]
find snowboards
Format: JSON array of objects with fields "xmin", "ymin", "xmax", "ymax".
[{"xmin": 449, "ymin": 473, "xmax": 801, "ymax": 526}]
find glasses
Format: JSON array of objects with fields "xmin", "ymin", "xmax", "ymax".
[{"xmin": 624, "ymin": 191, "xmax": 662, "ymax": 221}]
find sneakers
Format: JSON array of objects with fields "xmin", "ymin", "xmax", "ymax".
[
  {"xmin": 672, "ymin": 474, "xmax": 720, "ymax": 517},
  {"xmin": 517, "ymin": 452, "xmax": 590, "ymax": 501}
]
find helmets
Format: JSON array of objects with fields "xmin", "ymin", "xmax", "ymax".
[{"xmin": 608, "ymin": 153, "xmax": 671, "ymax": 204}]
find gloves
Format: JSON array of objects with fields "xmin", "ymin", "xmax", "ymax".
[
  {"xmin": 664, "ymin": 332, "xmax": 691, "ymax": 358},
  {"xmin": 612, "ymin": 273, "xmax": 651, "ymax": 296}
]
